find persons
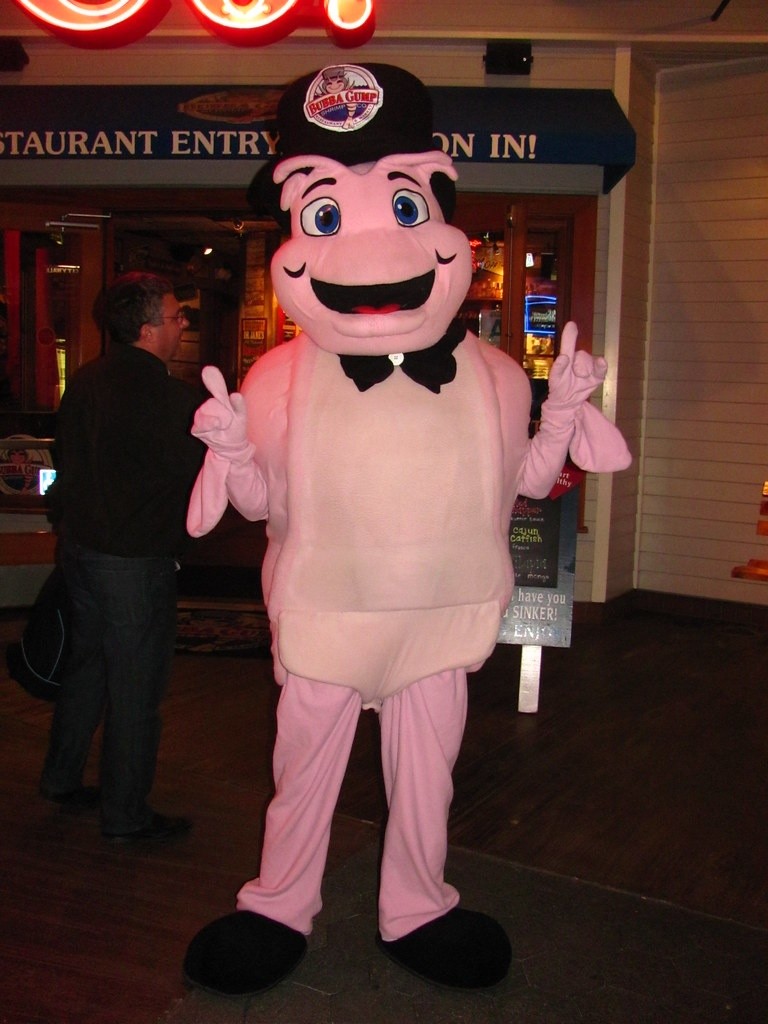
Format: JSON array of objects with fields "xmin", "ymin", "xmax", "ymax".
[{"xmin": 37, "ymin": 271, "xmax": 209, "ymax": 841}]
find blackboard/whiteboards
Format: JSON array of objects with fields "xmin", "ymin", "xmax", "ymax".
[{"xmin": 496, "ymin": 376, "xmax": 578, "ymax": 648}]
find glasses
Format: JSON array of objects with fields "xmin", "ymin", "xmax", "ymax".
[{"xmin": 153, "ymin": 311, "xmax": 184, "ymax": 322}]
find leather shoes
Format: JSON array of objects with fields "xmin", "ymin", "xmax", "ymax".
[
  {"xmin": 38, "ymin": 786, "xmax": 101, "ymax": 806},
  {"xmin": 101, "ymin": 812, "xmax": 193, "ymax": 840}
]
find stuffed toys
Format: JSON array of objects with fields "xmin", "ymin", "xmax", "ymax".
[{"xmin": 184, "ymin": 61, "xmax": 634, "ymax": 1003}]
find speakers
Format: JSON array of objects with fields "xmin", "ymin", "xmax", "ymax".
[{"xmin": 485, "ymin": 41, "xmax": 533, "ymax": 76}]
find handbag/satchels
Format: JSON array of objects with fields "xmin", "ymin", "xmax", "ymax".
[{"xmin": 6, "ymin": 568, "xmax": 72, "ymax": 701}]
